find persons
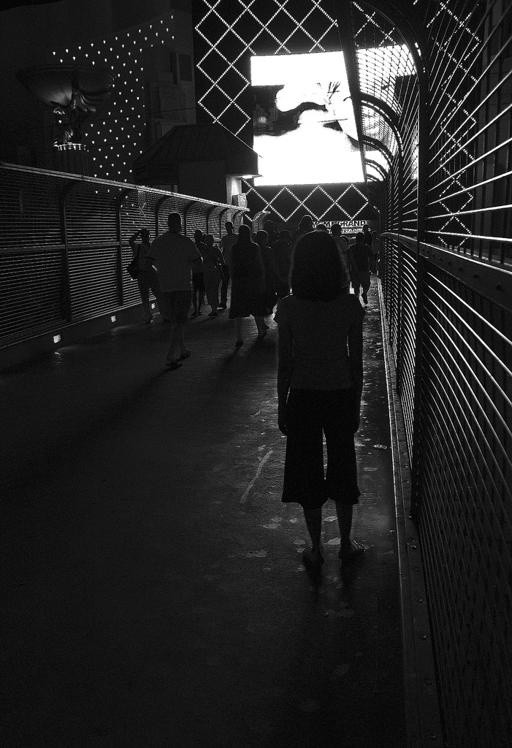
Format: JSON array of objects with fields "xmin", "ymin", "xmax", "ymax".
[
  {"xmin": 272, "ymin": 229, "xmax": 367, "ymax": 565},
  {"xmin": 129, "ymin": 211, "xmax": 380, "ymax": 368}
]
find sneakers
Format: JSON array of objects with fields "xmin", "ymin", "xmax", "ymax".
[
  {"xmin": 338, "ymin": 540, "xmax": 364, "ymax": 559},
  {"xmin": 302, "ymin": 547, "xmax": 325, "ymax": 565}
]
